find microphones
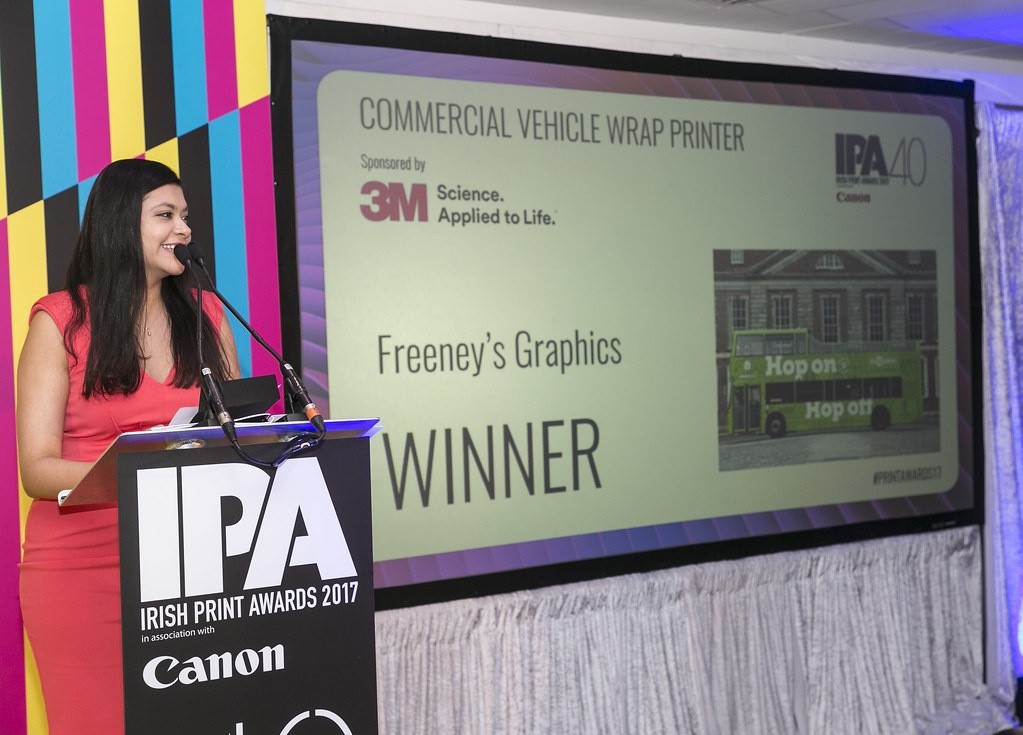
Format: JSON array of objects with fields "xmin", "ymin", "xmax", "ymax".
[
  {"xmin": 187, "ymin": 241, "xmax": 326, "ymax": 432},
  {"xmin": 174, "ymin": 243, "xmax": 237, "ymax": 444}
]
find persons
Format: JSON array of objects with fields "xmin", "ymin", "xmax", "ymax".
[{"xmin": 17, "ymin": 159, "xmax": 240, "ymax": 734}]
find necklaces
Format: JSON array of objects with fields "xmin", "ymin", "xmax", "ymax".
[{"xmin": 141, "ymin": 325, "xmax": 151, "ymax": 336}]
